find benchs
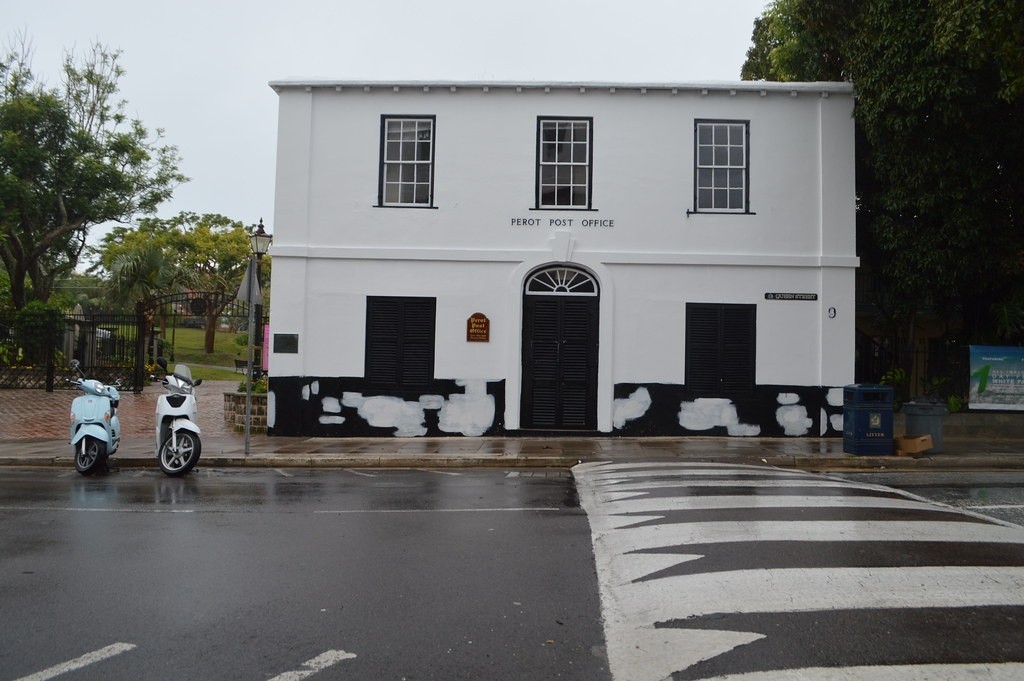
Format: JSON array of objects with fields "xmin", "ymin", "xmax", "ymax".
[{"xmin": 234, "ymin": 358, "xmax": 254, "ymax": 374}]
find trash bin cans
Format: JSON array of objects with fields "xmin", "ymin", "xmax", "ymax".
[
  {"xmin": 843, "ymin": 384, "xmax": 895, "ymax": 457},
  {"xmin": 902, "ymin": 403, "xmax": 945, "ymax": 455}
]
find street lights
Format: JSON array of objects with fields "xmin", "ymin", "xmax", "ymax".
[{"xmin": 245, "ymin": 217, "xmax": 273, "ymax": 453}]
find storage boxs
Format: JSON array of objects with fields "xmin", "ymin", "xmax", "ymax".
[{"xmin": 895, "ymin": 434, "xmax": 933, "ymax": 457}]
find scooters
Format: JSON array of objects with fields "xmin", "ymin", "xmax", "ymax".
[
  {"xmin": 154, "ymin": 356, "xmax": 204, "ymax": 478},
  {"xmin": 65, "ymin": 359, "xmax": 123, "ymax": 475}
]
